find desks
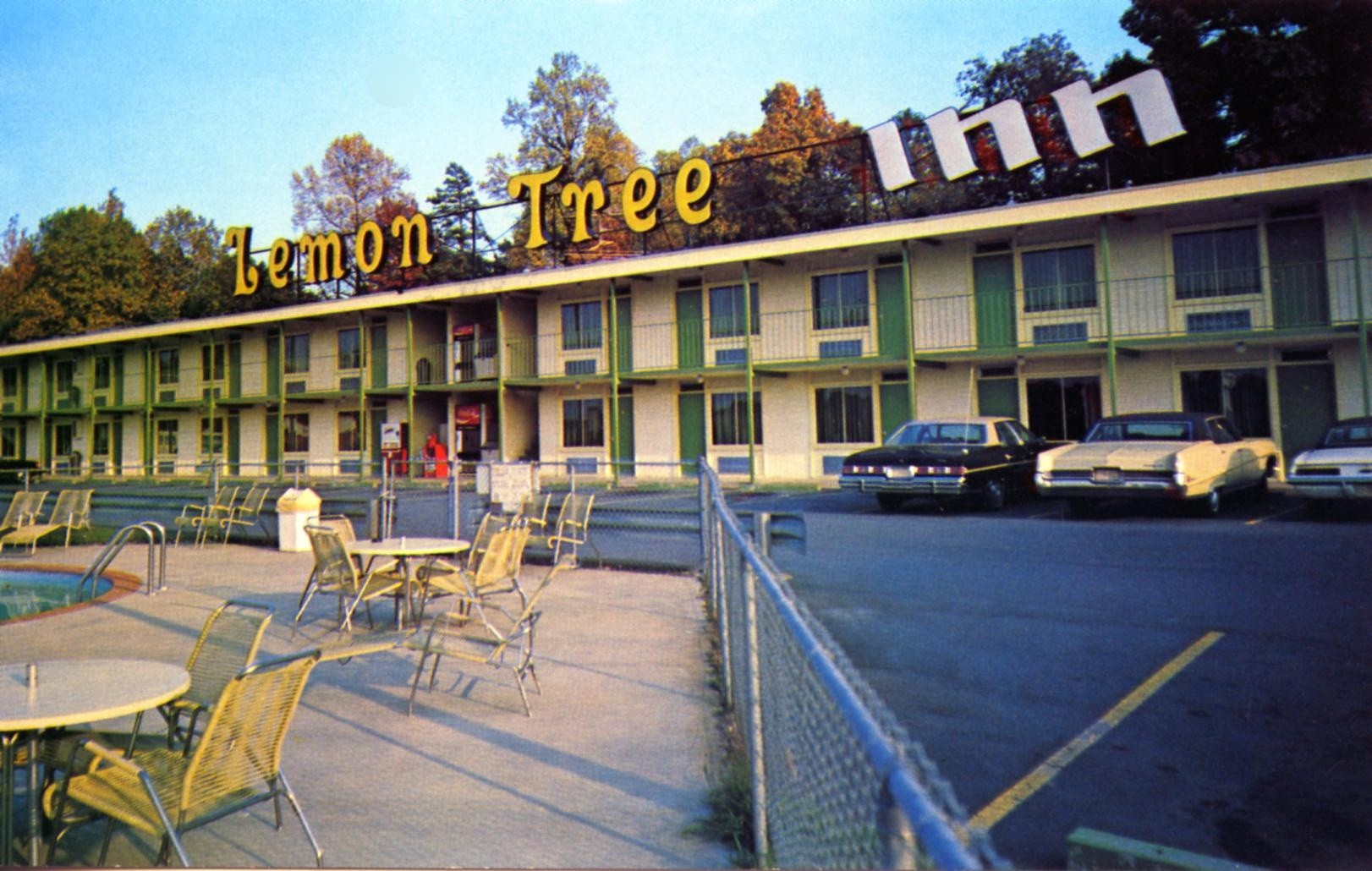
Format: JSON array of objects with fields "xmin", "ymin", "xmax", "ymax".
[
  {"xmin": 0, "ymin": 660, "xmax": 191, "ymax": 868},
  {"xmin": 346, "ymin": 538, "xmax": 471, "ymax": 633}
]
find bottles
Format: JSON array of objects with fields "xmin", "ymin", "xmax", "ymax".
[
  {"xmin": 400, "ymin": 535, "xmax": 406, "ymax": 546},
  {"xmin": 26, "ymin": 664, "xmax": 37, "ymax": 687}
]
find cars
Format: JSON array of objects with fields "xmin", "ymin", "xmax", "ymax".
[
  {"xmin": 837, "ymin": 416, "xmax": 1048, "ymax": 511},
  {"xmin": 0, "ymin": 460, "xmax": 40, "ymax": 484},
  {"xmin": 1033, "ymin": 411, "xmax": 1285, "ymax": 521},
  {"xmin": 1285, "ymin": 417, "xmax": 1372, "ymax": 511}
]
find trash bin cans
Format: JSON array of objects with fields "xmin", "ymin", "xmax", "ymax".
[{"xmin": 275, "ymin": 486, "xmax": 322, "ymax": 553}]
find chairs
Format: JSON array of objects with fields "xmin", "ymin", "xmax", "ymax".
[{"xmin": 0, "ymin": 484, "xmax": 595, "ymax": 868}]
